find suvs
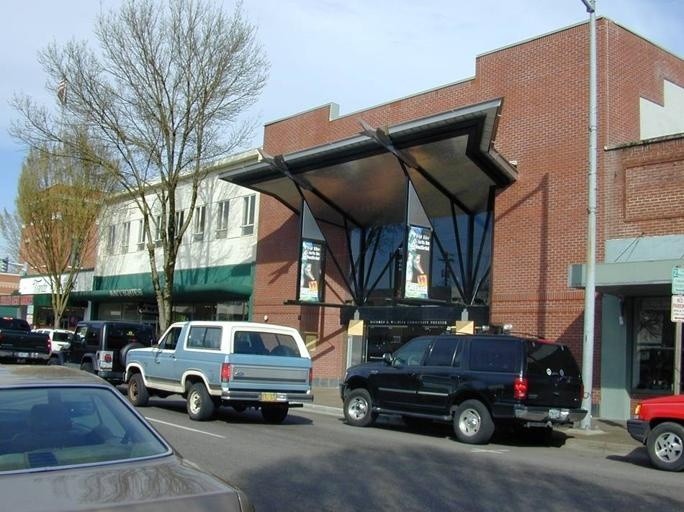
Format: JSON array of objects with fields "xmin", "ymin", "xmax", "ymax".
[
  {"xmin": 123, "ymin": 319, "xmax": 313, "ymax": 422},
  {"xmin": 49, "ymin": 320, "xmax": 158, "ymax": 386},
  {"xmin": 340, "ymin": 332, "xmax": 588, "ymax": 444}
]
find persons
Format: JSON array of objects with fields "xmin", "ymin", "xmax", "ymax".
[
  {"xmin": 303, "ymin": 258, "xmax": 321, "ymax": 288},
  {"xmin": 410, "ymin": 249, "xmax": 430, "ymax": 285}
]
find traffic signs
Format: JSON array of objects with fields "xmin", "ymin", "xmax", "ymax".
[{"xmin": 671, "ymin": 295, "xmax": 684, "ymax": 321}]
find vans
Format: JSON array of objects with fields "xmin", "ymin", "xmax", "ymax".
[{"xmin": 31, "ymin": 328, "xmax": 82, "ymax": 356}]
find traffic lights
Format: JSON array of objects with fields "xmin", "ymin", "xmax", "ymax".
[{"xmin": 2, "ymin": 258, "xmax": 7, "ymax": 272}]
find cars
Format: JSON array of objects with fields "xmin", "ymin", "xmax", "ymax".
[
  {"xmin": 0, "ymin": 361, "xmax": 251, "ymax": 512},
  {"xmin": 627, "ymin": 395, "xmax": 684, "ymax": 473}
]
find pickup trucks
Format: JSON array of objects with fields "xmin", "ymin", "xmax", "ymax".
[{"xmin": 0, "ymin": 317, "xmax": 51, "ymax": 363}]
